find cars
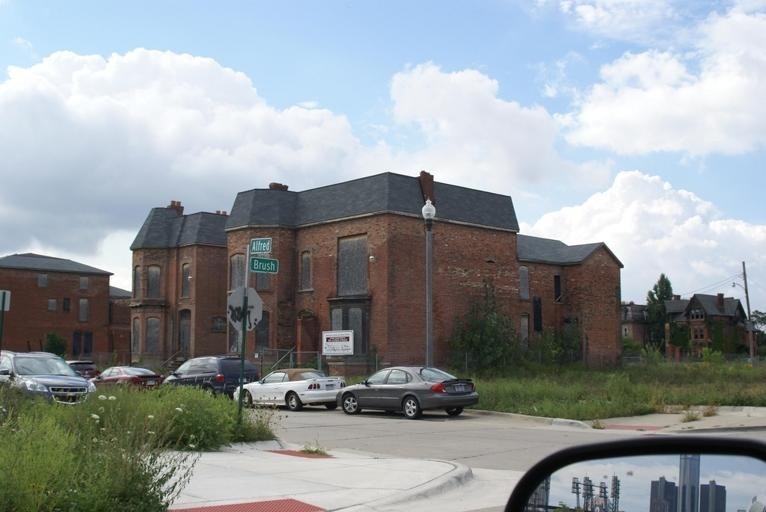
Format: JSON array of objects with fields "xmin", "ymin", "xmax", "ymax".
[
  {"xmin": 64, "ymin": 357, "xmax": 101, "ymax": 379},
  {"xmin": 89, "ymin": 364, "xmax": 164, "ymax": 390},
  {"xmin": 335, "ymin": 365, "xmax": 480, "ymax": 420}
]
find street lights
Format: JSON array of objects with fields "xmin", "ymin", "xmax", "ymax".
[
  {"xmin": 732, "ymin": 281, "xmax": 755, "ymax": 367},
  {"xmin": 420, "ymin": 195, "xmax": 438, "ymax": 370}
]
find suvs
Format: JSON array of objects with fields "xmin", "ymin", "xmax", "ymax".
[
  {"xmin": 160, "ymin": 352, "xmax": 262, "ymax": 398},
  {"xmin": 0, "ymin": 348, "xmax": 97, "ymax": 407}
]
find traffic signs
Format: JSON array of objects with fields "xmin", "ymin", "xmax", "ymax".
[
  {"xmin": 249, "ymin": 237, "xmax": 273, "ymax": 255},
  {"xmin": 250, "ymin": 257, "xmax": 279, "ymax": 274}
]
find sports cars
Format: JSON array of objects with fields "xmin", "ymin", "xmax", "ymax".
[{"xmin": 230, "ymin": 366, "xmax": 348, "ymax": 412}]
